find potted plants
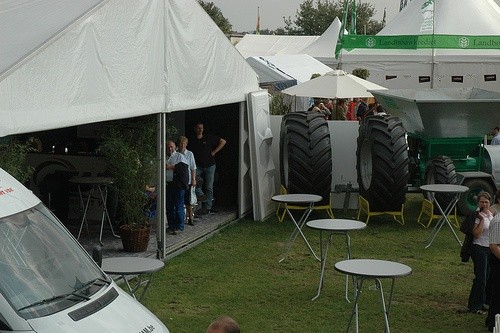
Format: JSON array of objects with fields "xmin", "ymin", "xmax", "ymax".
[{"xmin": 95, "ymin": 112, "xmax": 180, "ymax": 252}]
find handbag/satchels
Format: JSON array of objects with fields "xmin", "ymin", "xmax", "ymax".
[{"xmin": 189, "ymin": 186, "xmax": 198, "ymax": 206}]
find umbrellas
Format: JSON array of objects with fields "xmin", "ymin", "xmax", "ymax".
[{"xmin": 280, "ymin": 70, "xmax": 389, "ymax": 120}]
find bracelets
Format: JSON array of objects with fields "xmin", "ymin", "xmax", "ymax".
[{"xmin": 192, "ymin": 179, "xmax": 196, "ymax": 181}]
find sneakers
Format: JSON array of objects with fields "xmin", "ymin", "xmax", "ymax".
[
  {"xmin": 173, "ymin": 228, "xmax": 182, "ymax": 235},
  {"xmin": 166, "ymin": 225, "xmax": 173, "ymax": 232},
  {"xmin": 471, "ymin": 306, "xmax": 489, "ymax": 315},
  {"xmin": 206, "ymin": 209, "xmax": 215, "ymax": 215}
]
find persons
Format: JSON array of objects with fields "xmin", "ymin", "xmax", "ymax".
[
  {"xmin": 490, "ymin": 126, "xmax": 500, "ymax": 145},
  {"xmin": 206, "ymin": 316, "xmax": 240, "ymax": 333},
  {"xmin": 133, "ymin": 121, "xmax": 227, "ymax": 235},
  {"xmin": 308, "ymin": 97, "xmax": 384, "ymax": 123},
  {"xmin": 460, "ymin": 191, "xmax": 500, "ymax": 331}
]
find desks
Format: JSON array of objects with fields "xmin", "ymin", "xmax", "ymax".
[
  {"xmin": 102, "ymin": 257, "xmax": 165, "ymax": 304},
  {"xmin": 420, "ymin": 184, "xmax": 471, "ymax": 249},
  {"xmin": 302, "ymin": 219, "xmax": 368, "ymax": 304},
  {"xmin": 68, "ymin": 176, "xmax": 123, "ymax": 247},
  {"xmin": 272, "ymin": 194, "xmax": 324, "ymax": 262},
  {"xmin": 334, "ymin": 260, "xmax": 413, "ymax": 333}
]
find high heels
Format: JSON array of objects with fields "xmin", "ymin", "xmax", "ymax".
[{"xmin": 188, "ymin": 219, "xmax": 195, "ymax": 226}]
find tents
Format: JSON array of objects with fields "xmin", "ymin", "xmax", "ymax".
[{"xmin": 0, "ymin": 1, "xmax": 500, "ymax": 218}]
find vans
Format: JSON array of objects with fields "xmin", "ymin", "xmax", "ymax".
[{"xmin": 0, "ymin": 167, "xmax": 171, "ymax": 333}]
